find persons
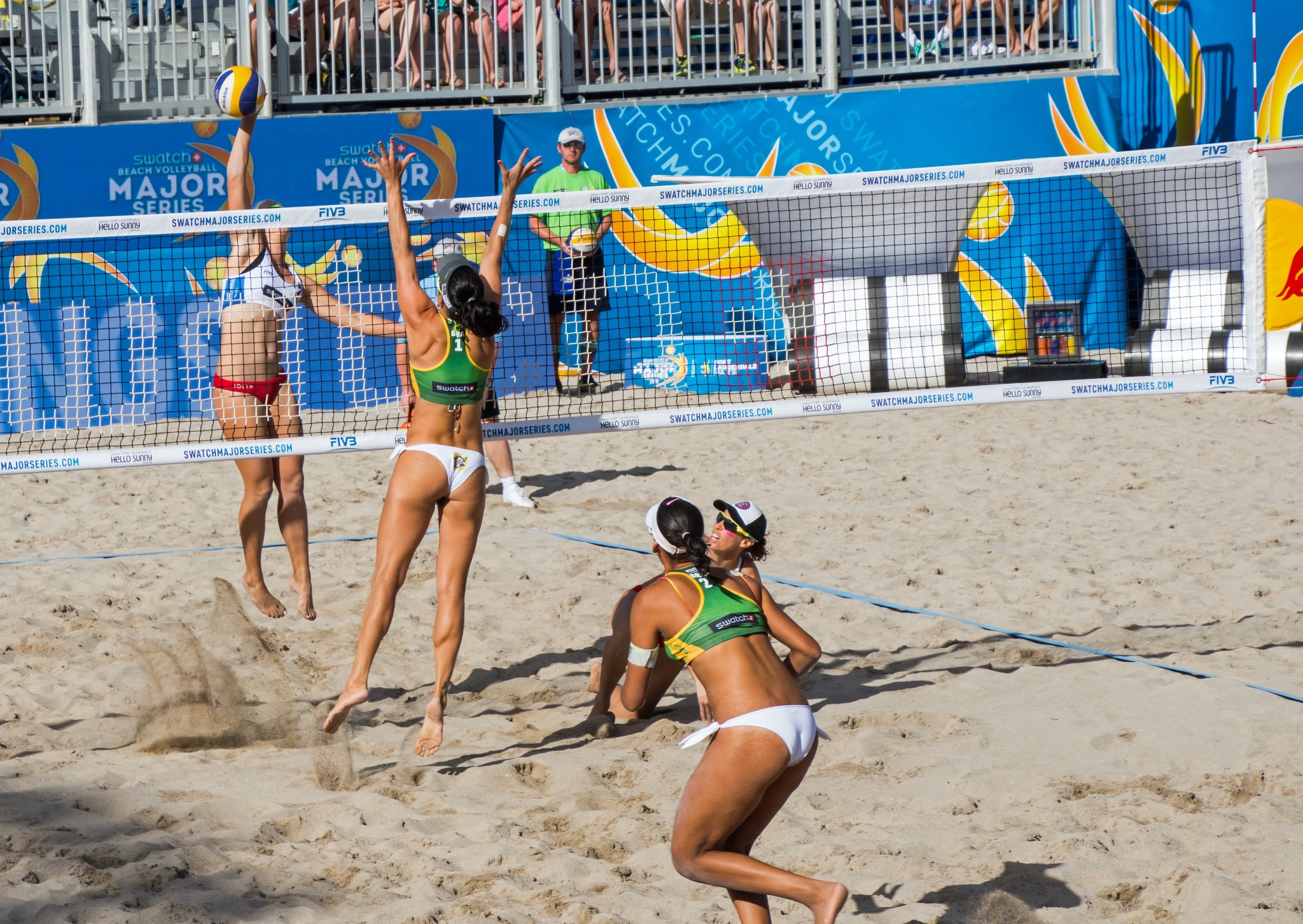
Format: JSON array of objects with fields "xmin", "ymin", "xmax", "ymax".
[
  {"xmin": 250, "ymin": 0, "xmax": 627, "ymax": 93},
  {"xmin": 661, "ymin": 0, "xmax": 787, "ymax": 76},
  {"xmin": 126, "ymin": 0, "xmax": 189, "ymax": 27},
  {"xmin": 979, "ymin": 0, "xmax": 1064, "ymax": 54},
  {"xmin": 322, "ymin": 138, "xmax": 543, "ymax": 759},
  {"xmin": 620, "ymin": 496, "xmax": 850, "ymax": 924},
  {"xmin": 213, "ymin": 94, "xmax": 408, "ymax": 618},
  {"xmin": 394, "ymin": 238, "xmax": 538, "ymax": 508},
  {"xmin": 529, "ymin": 126, "xmax": 620, "ymax": 394},
  {"xmin": 582, "ymin": 500, "xmax": 770, "ymax": 735},
  {"xmin": 880, "ymin": 0, "xmax": 976, "ymax": 62}
]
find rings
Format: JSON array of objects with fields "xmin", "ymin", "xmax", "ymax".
[{"xmin": 513, "ymin": 7, "xmax": 515, "ymax": 8}]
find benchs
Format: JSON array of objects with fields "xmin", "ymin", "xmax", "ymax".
[{"xmin": 0, "ymin": 0, "xmax": 1069, "ymax": 108}]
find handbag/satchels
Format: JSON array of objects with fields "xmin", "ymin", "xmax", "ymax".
[
  {"xmin": 322, "ymin": 65, "xmax": 373, "ymax": 94},
  {"xmin": 0, "ymin": 69, "xmax": 56, "ymax": 105}
]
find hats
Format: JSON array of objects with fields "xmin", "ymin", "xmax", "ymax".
[
  {"xmin": 558, "ymin": 126, "xmax": 585, "ymax": 144},
  {"xmin": 435, "ymin": 237, "xmax": 463, "ymax": 259},
  {"xmin": 645, "ymin": 495, "xmax": 705, "ymax": 555},
  {"xmin": 713, "ymin": 499, "xmax": 767, "ymax": 540},
  {"xmin": 436, "ymin": 253, "xmax": 475, "ymax": 313}
]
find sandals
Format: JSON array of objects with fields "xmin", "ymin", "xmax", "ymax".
[{"xmin": 765, "ymin": 59, "xmax": 786, "ymax": 70}]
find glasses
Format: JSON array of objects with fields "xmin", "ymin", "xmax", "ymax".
[
  {"xmin": 716, "ymin": 511, "xmax": 759, "ymax": 543},
  {"xmin": 559, "ymin": 142, "xmax": 584, "ymax": 149}
]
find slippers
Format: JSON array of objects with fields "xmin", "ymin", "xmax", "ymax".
[
  {"xmin": 444, "ymin": 75, "xmax": 464, "ymax": 87},
  {"xmin": 611, "ymin": 73, "xmax": 628, "ymax": 83},
  {"xmin": 537, "ymin": 48, "xmax": 546, "ymax": 81},
  {"xmin": 486, "ymin": 74, "xmax": 504, "ymax": 88},
  {"xmin": 582, "ymin": 72, "xmax": 601, "ymax": 84}
]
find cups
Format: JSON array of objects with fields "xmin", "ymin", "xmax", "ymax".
[
  {"xmin": 561, "ymin": 97, "xmax": 564, "ymax": 104},
  {"xmin": 192, "ymin": 30, "xmax": 200, "ymax": 42},
  {"xmin": 538, "ymin": 98, "xmax": 544, "ymax": 104},
  {"xmin": 224, "ymin": 34, "xmax": 235, "ymax": 58},
  {"xmin": 569, "ymin": 95, "xmax": 585, "ymax": 103}
]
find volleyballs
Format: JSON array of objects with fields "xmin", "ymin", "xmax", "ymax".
[{"xmin": 214, "ymin": 65, "xmax": 266, "ymax": 118}]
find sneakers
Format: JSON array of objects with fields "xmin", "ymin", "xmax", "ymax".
[
  {"xmin": 913, "ymin": 41, "xmax": 944, "ymax": 61},
  {"xmin": 320, "ymin": 49, "xmax": 332, "ymax": 71},
  {"xmin": 962, "ymin": 36, "xmax": 1007, "ymax": 56},
  {"xmin": 502, "ymin": 486, "xmax": 535, "ymax": 508},
  {"xmin": 734, "ymin": 52, "xmax": 756, "ymax": 73},
  {"xmin": 676, "ymin": 55, "xmax": 688, "ymax": 76},
  {"xmin": 555, "ymin": 377, "xmax": 600, "ymax": 392}
]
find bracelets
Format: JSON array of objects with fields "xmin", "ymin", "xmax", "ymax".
[
  {"xmin": 458, "ymin": 0, "xmax": 463, "ymax": 8},
  {"xmin": 397, "ymin": 1, "xmax": 402, "ymax": 8}
]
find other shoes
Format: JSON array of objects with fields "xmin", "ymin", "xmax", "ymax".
[
  {"xmin": 424, "ymin": 77, "xmax": 446, "ymax": 87},
  {"xmin": 126, "ymin": 13, "xmax": 147, "ymax": 26},
  {"xmin": 307, "ymin": 72, "xmax": 325, "ymax": 92},
  {"xmin": 166, "ymin": 12, "xmax": 188, "ymax": 25}
]
